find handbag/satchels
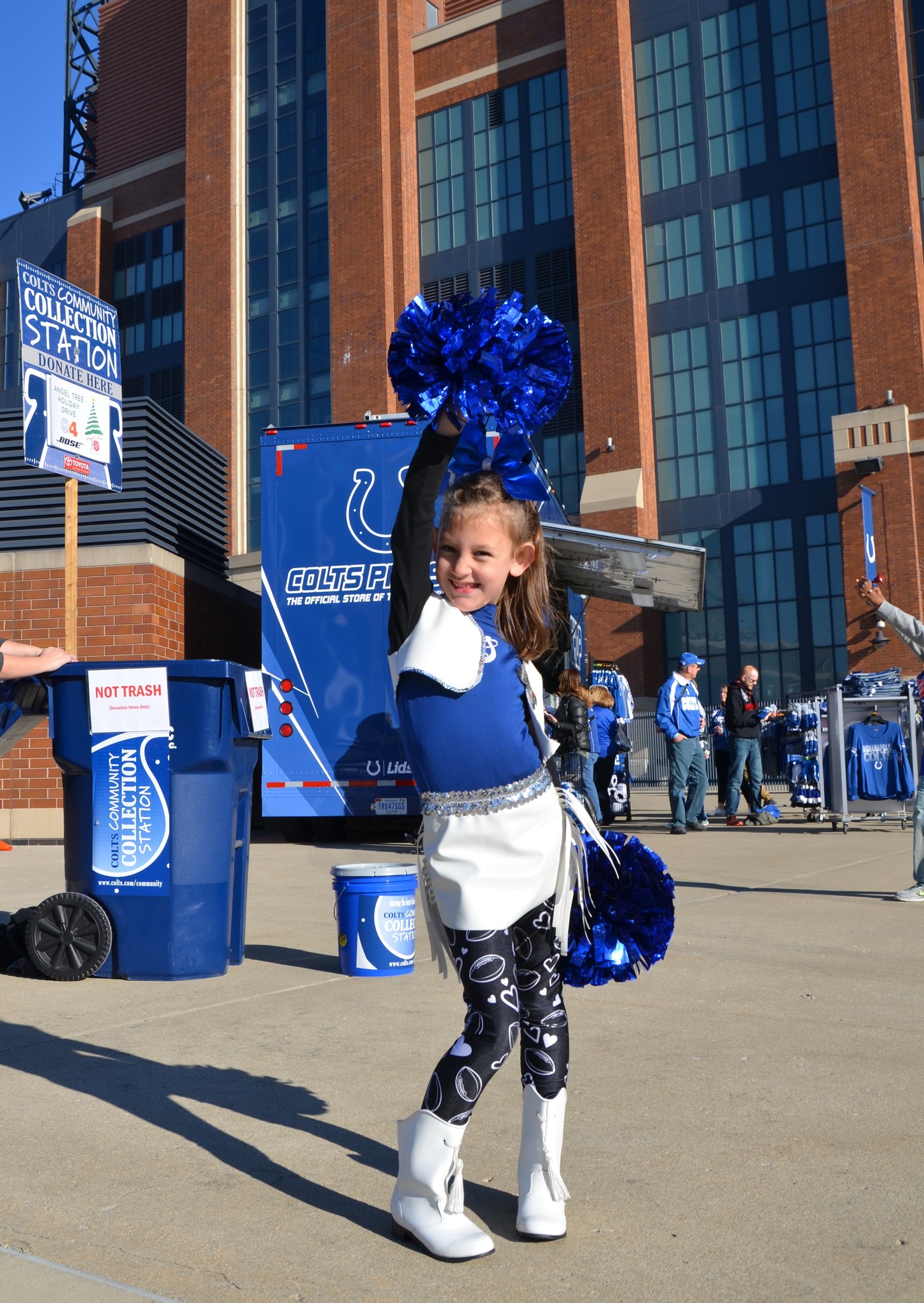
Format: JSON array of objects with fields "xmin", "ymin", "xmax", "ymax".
[{"xmin": 608, "ymin": 708, "xmax": 631, "ymax": 755}]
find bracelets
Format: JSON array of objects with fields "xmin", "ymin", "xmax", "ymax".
[{"xmin": 673, "ymin": 732, "xmax": 679, "ymax": 739}]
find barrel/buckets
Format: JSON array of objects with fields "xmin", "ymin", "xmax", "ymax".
[{"xmin": 332, "ymin": 858, "xmax": 420, "ymax": 978}]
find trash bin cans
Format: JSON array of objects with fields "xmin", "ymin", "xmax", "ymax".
[{"xmin": 22, "ymin": 657, "xmax": 274, "ymax": 983}]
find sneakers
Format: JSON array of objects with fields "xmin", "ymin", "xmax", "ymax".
[
  {"xmin": 707, "ymin": 808, "xmax": 725, "ymax": 816},
  {"xmin": 894, "ymin": 882, "xmax": 924, "ymax": 901},
  {"xmin": 726, "ymin": 814, "xmax": 744, "ymax": 826},
  {"xmin": 747, "ymin": 807, "xmax": 751, "ymax": 814},
  {"xmin": 697, "ymin": 819, "xmax": 709, "ymax": 826},
  {"xmin": 664, "ymin": 822, "xmax": 673, "ymax": 828}
]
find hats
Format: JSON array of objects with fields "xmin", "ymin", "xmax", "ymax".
[
  {"xmin": 785, "ymin": 703, "xmax": 821, "ymax": 807},
  {"xmin": 755, "ymin": 804, "xmax": 781, "ymax": 819},
  {"xmin": 677, "ymin": 652, "xmax": 706, "ymax": 667},
  {"xmin": 758, "ymin": 704, "xmax": 777, "ymax": 720}
]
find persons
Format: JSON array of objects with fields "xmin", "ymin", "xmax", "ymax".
[
  {"xmin": 706, "ymin": 684, "xmax": 753, "ymax": 818},
  {"xmin": 541, "ymin": 670, "xmax": 602, "ymax": 837},
  {"xmin": 855, "ymin": 574, "xmax": 924, "ymax": 903},
  {"xmin": 387, "ymin": 382, "xmax": 622, "ymax": 1262},
  {"xmin": 576, "ymin": 686, "xmax": 605, "ymax": 826},
  {"xmin": 587, "ymin": 684, "xmax": 619, "ymax": 827},
  {"xmin": 656, "ymin": 679, "xmax": 711, "ymax": 829},
  {"xmin": 724, "ymin": 665, "xmax": 777, "ymax": 827},
  {"xmin": 654, "ymin": 653, "xmax": 709, "ymax": 835}
]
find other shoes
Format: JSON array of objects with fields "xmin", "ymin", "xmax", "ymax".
[{"xmin": 762, "ymin": 798, "xmax": 777, "ymax": 807}]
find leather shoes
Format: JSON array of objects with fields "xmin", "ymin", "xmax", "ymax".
[
  {"xmin": 670, "ymin": 825, "xmax": 687, "ymax": 834},
  {"xmin": 685, "ymin": 822, "xmax": 707, "ymax": 831}
]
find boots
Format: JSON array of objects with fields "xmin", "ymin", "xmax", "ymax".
[
  {"xmin": 390, "ymin": 1109, "xmax": 496, "ymax": 1264},
  {"xmin": 514, "ymin": 1083, "xmax": 571, "ymax": 1241}
]
